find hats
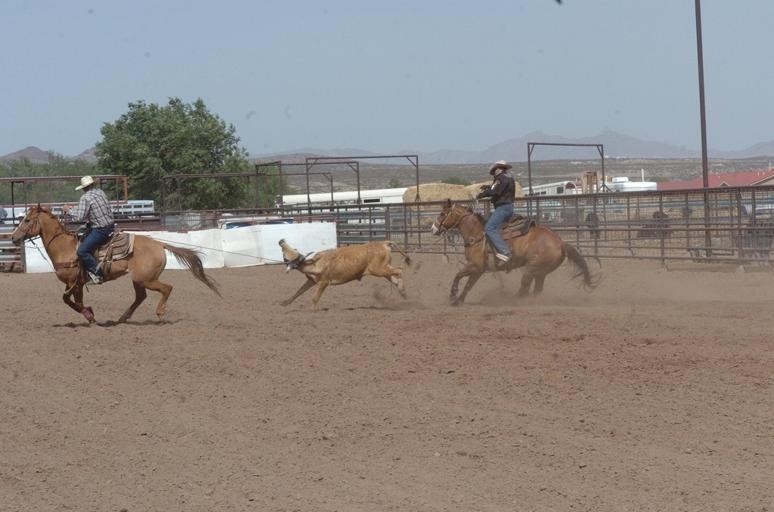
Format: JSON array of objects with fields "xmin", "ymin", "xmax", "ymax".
[
  {"xmin": 75, "ymin": 175, "xmax": 98, "ymax": 191},
  {"xmin": 489, "ymin": 160, "xmax": 512, "ymax": 175}
]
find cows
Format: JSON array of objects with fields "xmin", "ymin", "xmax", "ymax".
[{"xmin": 278, "ymin": 238, "xmax": 412, "ymax": 313}]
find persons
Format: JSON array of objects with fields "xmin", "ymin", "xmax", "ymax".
[
  {"xmin": 476, "ymin": 160, "xmax": 514, "ymax": 268},
  {"xmin": 61, "ymin": 175, "xmax": 113, "ymax": 284}
]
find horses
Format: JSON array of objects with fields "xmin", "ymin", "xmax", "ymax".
[
  {"xmin": 429, "ymin": 198, "xmax": 602, "ymax": 307},
  {"xmin": 10, "ymin": 202, "xmax": 224, "ymax": 324}
]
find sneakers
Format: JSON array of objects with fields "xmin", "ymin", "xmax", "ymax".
[{"xmin": 495, "ymin": 254, "xmax": 513, "ymax": 268}]
